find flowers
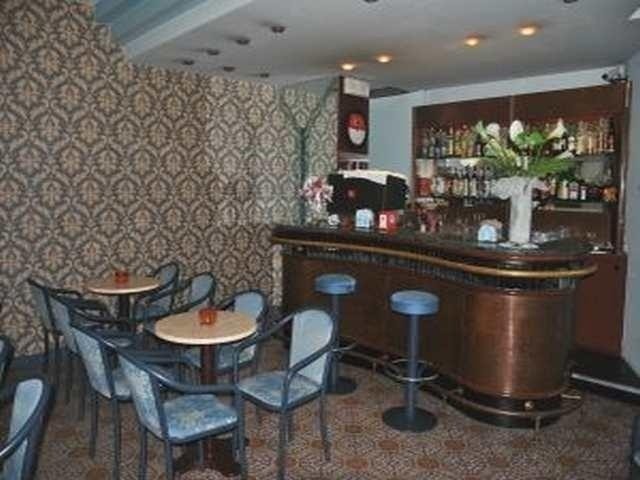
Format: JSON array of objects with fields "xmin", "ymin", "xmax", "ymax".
[
  {"xmin": 460, "ymin": 119, "xmax": 578, "ymax": 198},
  {"xmin": 298, "ymin": 176, "xmax": 333, "ymax": 214}
]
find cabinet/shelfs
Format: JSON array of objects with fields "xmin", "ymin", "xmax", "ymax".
[{"xmin": 410, "ymin": 77, "xmax": 632, "ymax": 359}]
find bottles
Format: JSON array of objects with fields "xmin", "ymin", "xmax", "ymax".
[
  {"xmin": 534, "ymin": 116, "xmax": 616, "ymax": 154},
  {"xmin": 419, "ymin": 121, "xmax": 482, "ymax": 156},
  {"xmin": 544, "ymin": 170, "xmax": 590, "ymax": 203},
  {"xmin": 431, "ymin": 163, "xmax": 498, "ymax": 198}
]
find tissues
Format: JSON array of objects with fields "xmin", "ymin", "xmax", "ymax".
[{"xmin": 477, "ymin": 218, "xmax": 503, "ymax": 243}]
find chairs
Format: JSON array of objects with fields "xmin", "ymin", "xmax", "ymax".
[
  {"xmin": 1, "ymin": 334, "xmax": 14, "ymax": 391},
  {"xmin": 0, "ymin": 373, "xmax": 51, "ymax": 480}
]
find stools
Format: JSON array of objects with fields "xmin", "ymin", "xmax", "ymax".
[
  {"xmin": 381, "ymin": 289, "xmax": 442, "ymax": 433},
  {"xmin": 316, "ymin": 273, "xmax": 358, "ymax": 394}
]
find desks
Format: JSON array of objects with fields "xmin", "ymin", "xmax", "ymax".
[{"xmin": 267, "ymin": 220, "xmax": 600, "ymax": 430}]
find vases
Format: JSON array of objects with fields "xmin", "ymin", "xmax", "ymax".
[
  {"xmin": 510, "ymin": 187, "xmax": 531, "ymax": 244},
  {"xmin": 306, "ymin": 200, "xmax": 327, "ymax": 227}
]
